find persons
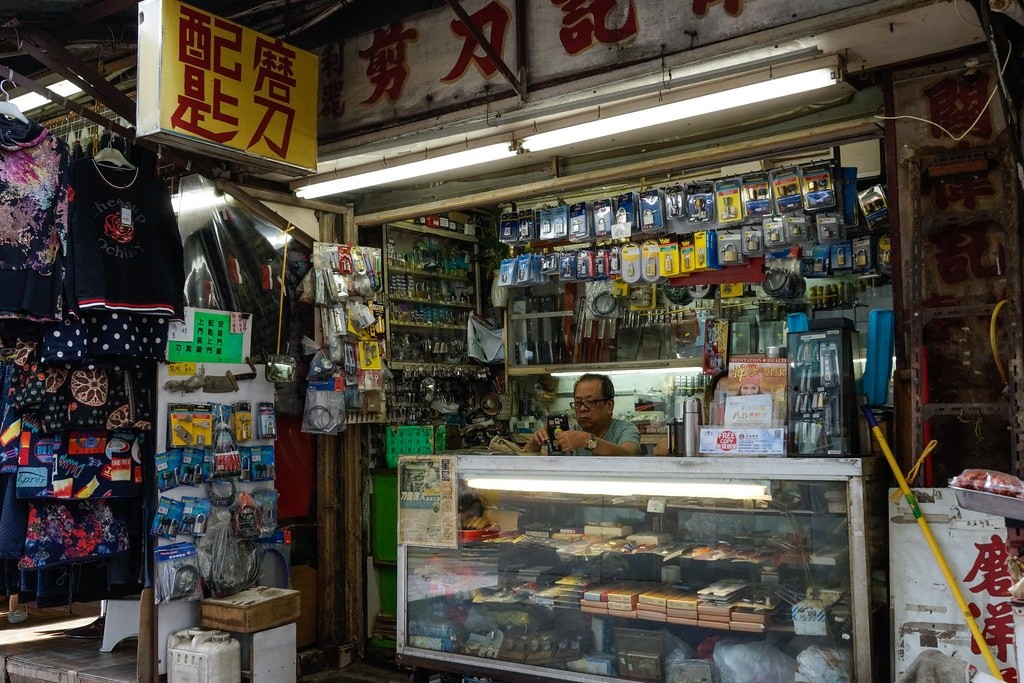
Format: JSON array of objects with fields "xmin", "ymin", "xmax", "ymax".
[
  {"xmin": 522, "ymin": 374, "xmax": 642, "ymax": 521},
  {"xmin": 420, "ymin": 462, "xmax": 437, "ymax": 490},
  {"xmin": 737, "ymin": 364, "xmax": 763, "ymax": 396}
]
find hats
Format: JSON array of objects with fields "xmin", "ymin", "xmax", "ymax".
[{"xmin": 740, "ymin": 364, "xmax": 760, "ymax": 388}]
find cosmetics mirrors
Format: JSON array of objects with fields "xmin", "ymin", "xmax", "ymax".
[{"xmin": 265, "ymin": 354, "xmax": 298, "ymax": 382}]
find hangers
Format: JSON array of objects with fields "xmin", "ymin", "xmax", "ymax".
[
  {"xmin": 0, "ymin": 78, "xmax": 29, "ymax": 125},
  {"xmin": 93, "ymin": 128, "xmax": 136, "ymax": 170}
]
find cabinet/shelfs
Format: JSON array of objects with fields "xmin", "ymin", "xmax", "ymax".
[
  {"xmin": 370, "ymin": 468, "xmax": 397, "ymax": 617},
  {"xmin": 393, "ymin": 454, "xmax": 873, "ymax": 683},
  {"xmin": 502, "ymin": 287, "xmax": 891, "ymax": 418},
  {"xmin": 381, "ymin": 219, "xmax": 482, "ymax": 427}
]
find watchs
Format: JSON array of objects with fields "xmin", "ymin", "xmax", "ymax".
[{"xmin": 583, "ymin": 433, "xmax": 598, "ymax": 451}]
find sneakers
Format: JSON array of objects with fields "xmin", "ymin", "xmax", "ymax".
[{"xmin": 64, "ymin": 617, "xmax": 105, "ymax": 638}]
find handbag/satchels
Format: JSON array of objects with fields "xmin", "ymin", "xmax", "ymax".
[{"xmin": 488, "ymin": 435, "xmax": 525, "ymax": 453}]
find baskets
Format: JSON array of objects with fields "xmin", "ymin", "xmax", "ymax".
[{"xmin": 386, "ymin": 425, "xmax": 445, "ymax": 468}]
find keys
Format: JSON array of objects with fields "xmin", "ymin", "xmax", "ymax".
[
  {"xmin": 181, "ymin": 516, "xmax": 195, "ymax": 532},
  {"xmin": 388, "ymin": 332, "xmax": 491, "ymax": 419},
  {"xmin": 162, "ymin": 466, "xmax": 194, "ymax": 487},
  {"xmin": 346, "ymin": 410, "xmax": 374, "ymax": 423},
  {"xmin": 157, "ymin": 518, "xmax": 172, "ymax": 534},
  {"xmin": 256, "ymin": 464, "xmax": 267, "ymax": 478}
]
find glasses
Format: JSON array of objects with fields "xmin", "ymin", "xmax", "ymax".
[{"xmin": 570, "ymin": 399, "xmax": 609, "ymax": 410}]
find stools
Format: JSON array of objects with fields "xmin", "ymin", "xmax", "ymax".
[{"xmin": 99, "ymin": 596, "xmax": 140, "ymax": 652}]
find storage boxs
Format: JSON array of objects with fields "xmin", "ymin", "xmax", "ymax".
[
  {"xmin": 396, "ymin": 449, "xmax": 890, "ymax": 683},
  {"xmin": 386, "ymin": 425, "xmax": 446, "ymax": 472},
  {"xmin": 630, "ymin": 411, "xmax": 675, "ymax": 455},
  {"xmin": 580, "ymin": 579, "xmax": 773, "ymax": 632},
  {"xmin": 201, "ymin": 585, "xmax": 302, "ymax": 634}
]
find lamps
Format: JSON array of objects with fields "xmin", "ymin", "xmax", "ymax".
[
  {"xmin": 460, "ymin": 475, "xmax": 773, "ymax": 501},
  {"xmin": 289, "ymin": 54, "xmax": 850, "ymax": 201}
]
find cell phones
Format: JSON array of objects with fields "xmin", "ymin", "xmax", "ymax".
[{"xmin": 547, "ymin": 414, "xmax": 570, "ymax": 452}]
